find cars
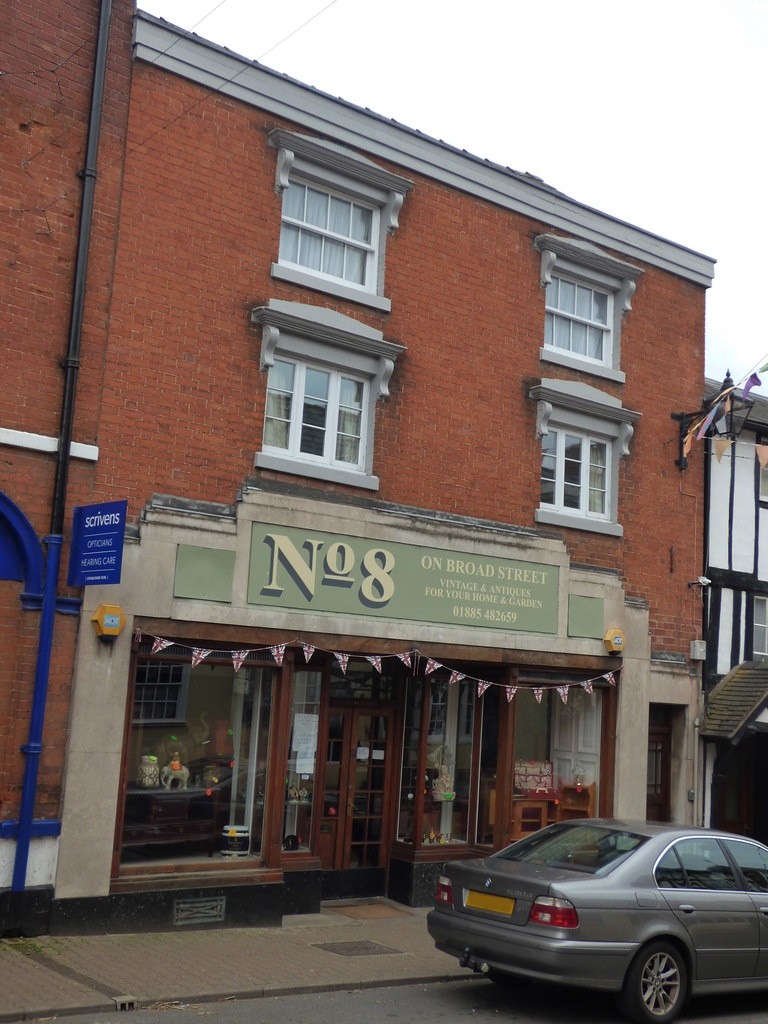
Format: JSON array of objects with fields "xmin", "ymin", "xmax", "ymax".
[
  {"xmin": 119, "ymin": 754, "xmax": 375, "ymax": 864},
  {"xmin": 427, "ymin": 818, "xmax": 768, "ymax": 1024},
  {"xmin": 353, "ymin": 767, "xmax": 500, "ymax": 857}
]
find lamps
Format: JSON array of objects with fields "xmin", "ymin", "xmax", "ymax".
[{"xmin": 672, "ymin": 369, "xmax": 755, "ymax": 470}]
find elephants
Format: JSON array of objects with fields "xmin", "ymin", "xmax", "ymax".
[{"xmin": 161, "ymin": 764, "xmax": 190, "ymax": 790}]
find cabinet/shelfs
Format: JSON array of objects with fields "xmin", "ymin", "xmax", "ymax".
[
  {"xmin": 511, "ymin": 800, "xmax": 550, "ymax": 839},
  {"xmin": 558, "ymin": 780, "xmax": 597, "ymax": 819}
]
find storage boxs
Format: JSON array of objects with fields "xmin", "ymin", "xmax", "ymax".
[{"xmin": 514, "ymin": 758, "xmax": 558, "ymax": 799}]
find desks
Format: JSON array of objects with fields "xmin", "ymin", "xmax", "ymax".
[{"xmin": 122, "ymin": 781, "xmax": 221, "ymax": 858}]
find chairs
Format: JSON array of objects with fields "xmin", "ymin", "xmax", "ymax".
[{"xmin": 682, "ymin": 854, "xmax": 717, "ymax": 887}]
